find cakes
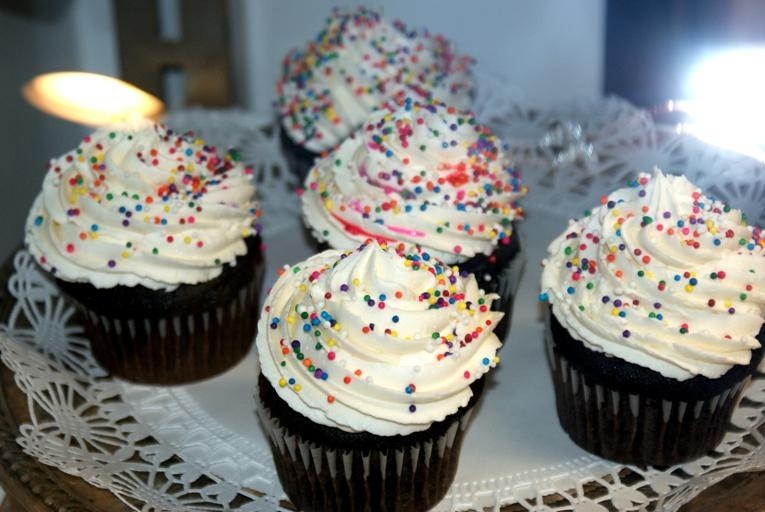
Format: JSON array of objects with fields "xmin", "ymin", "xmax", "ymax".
[
  {"xmin": 253, "ymin": 237, "xmax": 506, "ymax": 512},
  {"xmin": 298, "ymin": 102, "xmax": 529, "ymax": 343},
  {"xmin": 537, "ymin": 164, "xmax": 765, "ymax": 466},
  {"xmin": 275, "ymin": 5, "xmax": 477, "ymax": 182},
  {"xmin": 22, "ymin": 120, "xmax": 265, "ymax": 386}
]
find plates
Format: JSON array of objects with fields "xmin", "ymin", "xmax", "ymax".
[{"xmin": 0, "ymin": 93, "xmax": 761, "ymax": 511}]
